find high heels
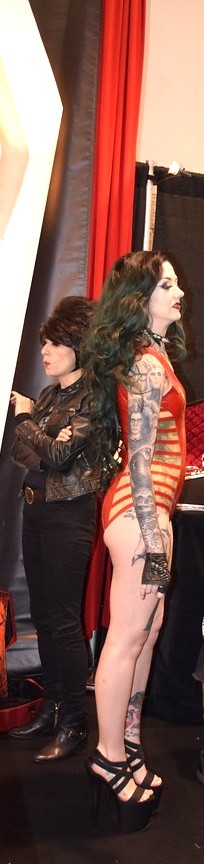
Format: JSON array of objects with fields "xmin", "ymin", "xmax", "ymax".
[
  {"xmin": 124, "ymin": 738, "xmax": 167, "ymax": 815},
  {"xmin": 85, "ymin": 747, "xmax": 156, "ymax": 833}
]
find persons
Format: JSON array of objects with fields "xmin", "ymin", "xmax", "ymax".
[
  {"xmin": 77, "ymin": 249, "xmax": 188, "ymax": 834},
  {"xmin": 7, "ymin": 295, "xmax": 101, "ymax": 765}
]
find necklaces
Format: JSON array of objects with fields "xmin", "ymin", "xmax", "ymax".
[{"xmin": 143, "ymin": 330, "xmax": 171, "ymax": 344}]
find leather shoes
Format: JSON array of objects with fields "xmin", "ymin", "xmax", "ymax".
[
  {"xmin": 7, "ymin": 701, "xmax": 63, "ymax": 738},
  {"xmin": 32, "ymin": 726, "xmax": 89, "ymax": 763}
]
